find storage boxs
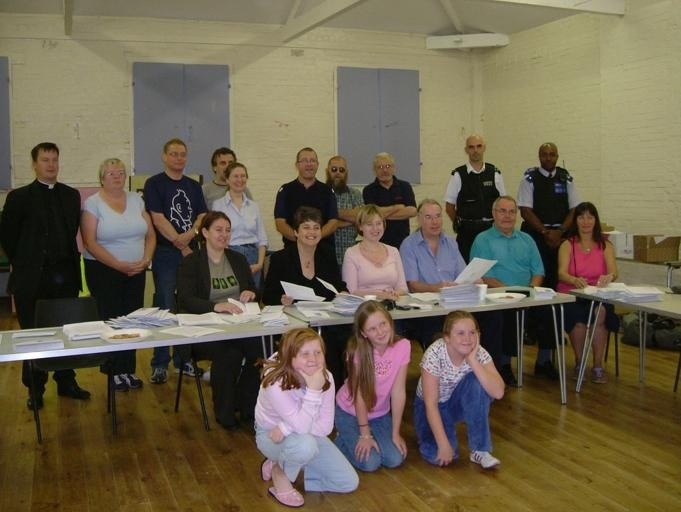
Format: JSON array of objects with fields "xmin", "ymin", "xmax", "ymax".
[
  {"xmin": 602, "ymin": 230, "xmax": 634, "ymax": 258},
  {"xmin": 633, "ymin": 233, "xmax": 681, "ymax": 263}
]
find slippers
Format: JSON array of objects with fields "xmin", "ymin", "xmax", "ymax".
[
  {"xmin": 261, "ymin": 455, "xmax": 275, "ymax": 481},
  {"xmin": 267, "ymin": 486, "xmax": 305, "ymax": 507}
]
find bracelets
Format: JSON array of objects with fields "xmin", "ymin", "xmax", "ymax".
[{"xmin": 359, "ymin": 423, "xmax": 369, "ymax": 427}]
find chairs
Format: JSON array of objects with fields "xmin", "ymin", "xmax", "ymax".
[
  {"xmin": 172, "ymin": 283, "xmax": 215, "ymax": 431},
  {"xmin": 26, "ymin": 294, "xmax": 120, "ymax": 444},
  {"xmin": 550, "ymin": 311, "xmax": 622, "ymax": 378}
]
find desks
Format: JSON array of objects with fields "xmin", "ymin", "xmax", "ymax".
[
  {"xmin": 615, "ymin": 256, "xmax": 681, "ymax": 289},
  {"xmin": 281, "ymin": 285, "xmax": 576, "ymax": 406},
  {"xmin": 569, "ymin": 281, "xmax": 681, "ymax": 393},
  {"xmin": 0, "ymin": 307, "xmax": 311, "ymax": 363}
]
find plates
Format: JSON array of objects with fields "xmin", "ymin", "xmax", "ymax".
[
  {"xmin": 101, "ymin": 327, "xmax": 152, "ymax": 343},
  {"xmin": 486, "ymin": 293, "xmax": 527, "ymax": 304}
]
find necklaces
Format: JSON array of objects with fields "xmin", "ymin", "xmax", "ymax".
[{"xmin": 587, "ymin": 248, "xmax": 590, "ymax": 251}]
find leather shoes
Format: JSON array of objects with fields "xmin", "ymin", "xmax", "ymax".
[
  {"xmin": 533, "ymin": 359, "xmax": 560, "ymax": 379},
  {"xmin": 56, "ymin": 385, "xmax": 91, "ymax": 400},
  {"xmin": 495, "ymin": 362, "xmax": 520, "ymax": 388},
  {"xmin": 28, "ymin": 390, "xmax": 44, "ymax": 409}
]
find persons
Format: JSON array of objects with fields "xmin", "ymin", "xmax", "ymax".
[
  {"xmin": 517, "ymin": 142, "xmax": 584, "ymax": 288},
  {"xmin": 262, "ymin": 207, "xmax": 346, "ymax": 394},
  {"xmin": 332, "ymin": 297, "xmax": 411, "ymax": 471},
  {"xmin": 444, "ymin": 136, "xmax": 506, "ymax": 266},
  {"xmin": 558, "ymin": 202, "xmax": 618, "ymax": 384},
  {"xmin": 254, "ymin": 324, "xmax": 359, "ymax": 506},
  {"xmin": 398, "ymin": 201, "xmax": 485, "ymax": 345},
  {"xmin": 470, "ymin": 196, "xmax": 559, "ymax": 386},
  {"xmin": 178, "ymin": 212, "xmax": 264, "ymax": 430},
  {"xmin": 275, "ymin": 148, "xmax": 418, "ymax": 265},
  {"xmin": 339, "ymin": 202, "xmax": 409, "ymax": 301},
  {"xmin": 0, "ymin": 138, "xmax": 269, "ymax": 410},
  {"xmin": 414, "ymin": 310, "xmax": 505, "ymax": 469}
]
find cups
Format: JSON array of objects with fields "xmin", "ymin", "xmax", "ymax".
[
  {"xmin": 365, "ymin": 294, "xmax": 377, "ymax": 300},
  {"xmin": 476, "ymin": 284, "xmax": 488, "ymax": 304}
]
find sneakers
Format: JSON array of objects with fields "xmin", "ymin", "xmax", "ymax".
[
  {"xmin": 469, "ymin": 450, "xmax": 499, "ymax": 471},
  {"xmin": 572, "ymin": 367, "xmax": 586, "ymax": 381},
  {"xmin": 121, "ymin": 372, "xmax": 143, "ymax": 388},
  {"xmin": 108, "ymin": 373, "xmax": 128, "ymax": 392},
  {"xmin": 175, "ymin": 360, "xmax": 204, "ymax": 377},
  {"xmin": 149, "ymin": 367, "xmax": 169, "ymax": 383},
  {"xmin": 591, "ymin": 367, "xmax": 607, "ymax": 383}
]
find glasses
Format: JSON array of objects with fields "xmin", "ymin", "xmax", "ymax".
[
  {"xmin": 99, "ymin": 170, "xmax": 126, "ymax": 178},
  {"xmin": 297, "ymin": 157, "xmax": 317, "ymax": 164},
  {"xmin": 377, "ymin": 163, "xmax": 393, "ymax": 170},
  {"xmin": 326, "ymin": 165, "xmax": 346, "ymax": 173}
]
